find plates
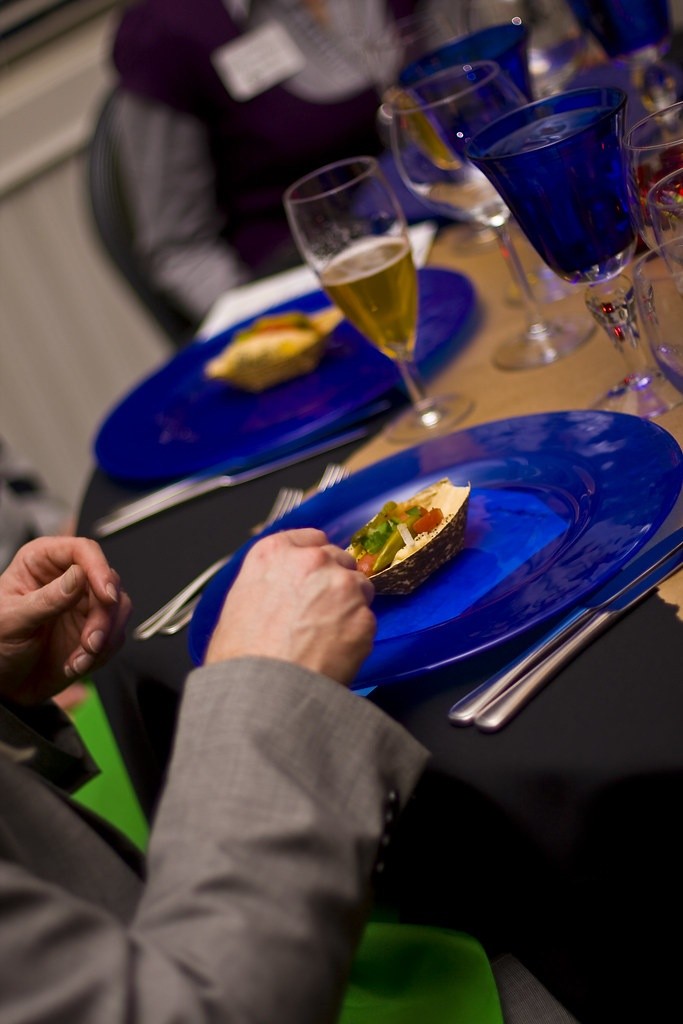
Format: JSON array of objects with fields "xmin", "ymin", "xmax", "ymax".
[
  {"xmin": 189, "ymin": 409, "xmax": 683, "ymax": 686},
  {"xmin": 93, "ymin": 269, "xmax": 474, "ymax": 476},
  {"xmin": 354, "ymin": 145, "xmax": 463, "ymax": 223}
]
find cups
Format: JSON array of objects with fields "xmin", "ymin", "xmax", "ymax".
[
  {"xmin": 622, "ymin": 101, "xmax": 683, "ymax": 265},
  {"xmin": 632, "ymin": 237, "xmax": 683, "ymax": 395},
  {"xmin": 648, "ymin": 168, "xmax": 683, "ymax": 296}
]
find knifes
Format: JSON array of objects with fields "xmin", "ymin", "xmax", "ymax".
[
  {"xmin": 448, "ymin": 526, "xmax": 683, "ymax": 728},
  {"xmin": 94, "ymin": 396, "xmax": 389, "ymax": 537}
]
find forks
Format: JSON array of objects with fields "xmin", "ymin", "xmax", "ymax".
[
  {"xmin": 132, "ymin": 487, "xmax": 304, "ymax": 639},
  {"xmin": 161, "ymin": 464, "xmax": 353, "ymax": 634}
]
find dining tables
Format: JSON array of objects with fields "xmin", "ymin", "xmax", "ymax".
[{"xmin": 76, "ymin": 42, "xmax": 681, "ymax": 1022}]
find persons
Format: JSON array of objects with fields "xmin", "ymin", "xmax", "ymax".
[
  {"xmin": 108, "ymin": 0, "xmax": 610, "ymax": 322},
  {"xmin": 0, "ymin": 529, "xmax": 683, "ymax": 1023}
]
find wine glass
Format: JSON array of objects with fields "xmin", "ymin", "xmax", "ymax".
[
  {"xmin": 284, "ymin": 157, "xmax": 474, "ymax": 442},
  {"xmin": 383, "ymin": 62, "xmax": 593, "ymax": 371},
  {"xmin": 397, "ymin": 23, "xmax": 535, "ymax": 167},
  {"xmin": 468, "ymin": 85, "xmax": 683, "ymax": 420},
  {"xmin": 568, "ymin": 0, "xmax": 683, "ymax": 131}
]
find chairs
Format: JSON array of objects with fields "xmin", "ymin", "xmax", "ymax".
[{"xmin": 82, "ymin": 80, "xmax": 249, "ymax": 343}]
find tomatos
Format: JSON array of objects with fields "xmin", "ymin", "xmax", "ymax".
[
  {"xmin": 355, "ymin": 552, "xmax": 383, "ymax": 576},
  {"xmin": 413, "ymin": 508, "xmax": 443, "ymax": 533}
]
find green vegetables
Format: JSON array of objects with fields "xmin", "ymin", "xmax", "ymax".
[{"xmin": 360, "ymin": 505, "xmax": 421, "ymax": 554}]
